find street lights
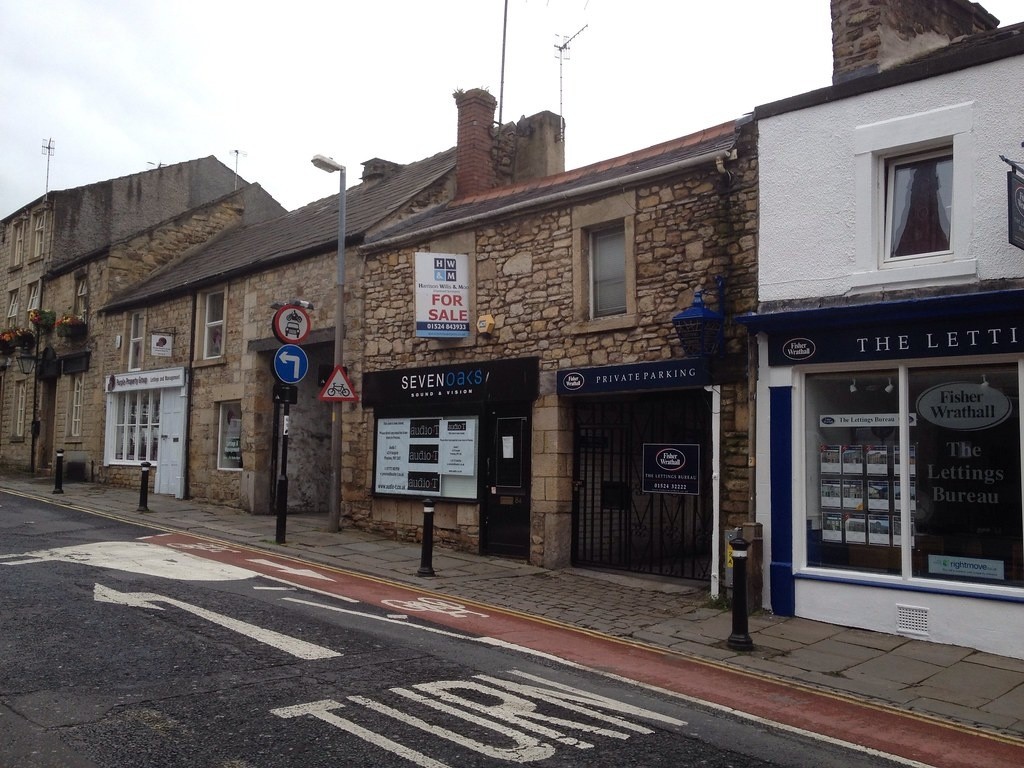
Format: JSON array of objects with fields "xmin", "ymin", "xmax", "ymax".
[
  {"xmin": 15, "ymin": 275, "xmax": 41, "ymax": 473},
  {"xmin": 311, "ymin": 154, "xmax": 347, "ymax": 533}
]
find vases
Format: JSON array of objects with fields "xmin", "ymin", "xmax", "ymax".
[{"xmin": 56, "ymin": 324, "xmax": 87, "ymax": 337}]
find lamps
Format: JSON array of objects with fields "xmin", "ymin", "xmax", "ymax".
[
  {"xmin": 850, "ymin": 378, "xmax": 856, "ymax": 392},
  {"xmin": 980, "ymin": 376, "xmax": 989, "ymax": 389},
  {"xmin": 672, "ymin": 274, "xmax": 727, "ymax": 358},
  {"xmin": 885, "ymin": 377, "xmax": 894, "ymax": 393}
]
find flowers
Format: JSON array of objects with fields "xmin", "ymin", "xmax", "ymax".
[
  {"xmin": 29, "ymin": 307, "xmax": 55, "ymax": 330},
  {"xmin": 0, "ymin": 325, "xmax": 35, "ymax": 347},
  {"xmin": 55, "ymin": 313, "xmax": 84, "ymax": 331}
]
